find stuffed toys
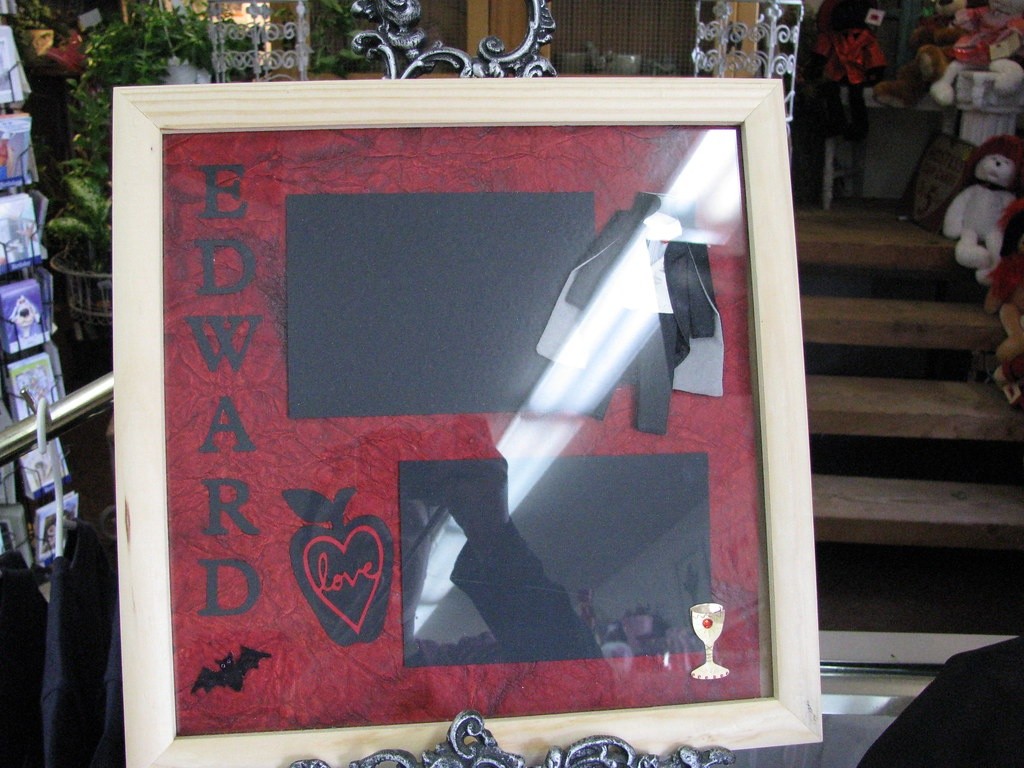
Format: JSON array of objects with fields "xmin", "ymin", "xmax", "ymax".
[
  {"xmin": 872, "ymin": 0, "xmax": 1024, "ymax": 111},
  {"xmin": 982, "ymin": 198, "xmax": 1024, "ymax": 411},
  {"xmin": 942, "ymin": 135, "xmax": 1024, "ymax": 286}
]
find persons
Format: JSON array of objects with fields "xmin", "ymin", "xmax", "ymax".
[{"xmin": 304, "ymin": 416, "xmax": 628, "ymax": 730}]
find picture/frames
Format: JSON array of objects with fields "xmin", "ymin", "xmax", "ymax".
[{"xmin": 112, "ymin": 77, "xmax": 825, "ymax": 768}]
